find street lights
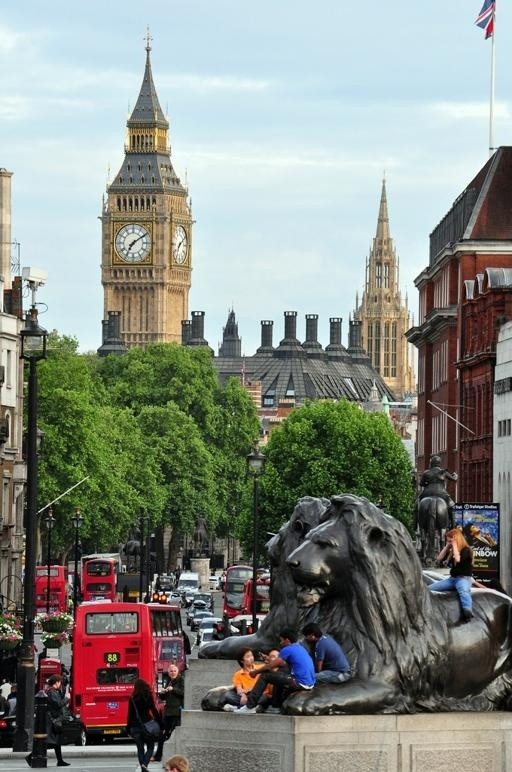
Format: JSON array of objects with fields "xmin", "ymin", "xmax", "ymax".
[
  {"xmin": 70, "ymin": 510, "xmax": 86, "ymax": 622},
  {"xmin": 245, "ymin": 446, "xmax": 266, "ymax": 635},
  {"xmin": 14, "ymin": 304, "xmax": 50, "ymax": 753},
  {"xmin": 44, "ymin": 506, "xmax": 57, "ymax": 613}
]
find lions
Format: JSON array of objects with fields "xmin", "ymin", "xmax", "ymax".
[{"xmin": 198, "ymin": 492, "xmax": 512, "ymax": 714}]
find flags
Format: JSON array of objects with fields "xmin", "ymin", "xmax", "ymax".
[{"xmin": 474, "ymin": 1, "xmax": 493, "ymax": 41}]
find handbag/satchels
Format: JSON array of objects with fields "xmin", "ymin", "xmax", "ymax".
[
  {"xmin": 143, "ymin": 720, "xmax": 160, "ymax": 736},
  {"xmin": 52, "ymin": 716, "xmax": 68, "ymax": 729}
]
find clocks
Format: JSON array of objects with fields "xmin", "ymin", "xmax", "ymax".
[
  {"xmin": 115, "ymin": 223, "xmax": 153, "ymax": 264},
  {"xmin": 174, "ymin": 225, "xmax": 190, "ymax": 266}
]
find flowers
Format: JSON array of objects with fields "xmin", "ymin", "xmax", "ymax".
[
  {"xmin": 32, "ymin": 611, "xmax": 74, "ymax": 628},
  {"xmin": 40, "ymin": 630, "xmax": 71, "ymax": 644},
  {"xmin": 0, "ymin": 611, "xmax": 23, "ymax": 640}
]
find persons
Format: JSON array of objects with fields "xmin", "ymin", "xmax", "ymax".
[
  {"xmin": 43, "ymin": 673, "xmax": 72, "ymax": 766},
  {"xmin": 426, "ymin": 527, "xmax": 475, "ymax": 623},
  {"xmin": 222, "ymin": 621, "xmax": 353, "ymax": 714},
  {"xmin": 126, "ymin": 678, "xmax": 164, "ymax": 772},
  {"xmin": 193, "ymin": 514, "xmax": 209, "ymax": 548},
  {"xmin": 417, "ymin": 455, "xmax": 458, "ymax": 508},
  {"xmin": 0, "ymin": 663, "xmax": 73, "ymax": 718},
  {"xmin": 149, "ymin": 664, "xmax": 185, "ymax": 761},
  {"xmin": 463, "ymin": 521, "xmax": 496, "ymax": 549},
  {"xmin": 161, "ymin": 753, "xmax": 190, "ymax": 772},
  {"xmin": 122, "ymin": 521, "xmax": 143, "ymax": 557}
]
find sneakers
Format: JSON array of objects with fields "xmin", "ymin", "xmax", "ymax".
[{"xmin": 223, "ymin": 704, "xmax": 263, "ymax": 714}]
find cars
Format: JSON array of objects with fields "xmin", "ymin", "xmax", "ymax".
[
  {"xmin": 0, "ymin": 696, "xmax": 87, "ymax": 748},
  {"xmin": 419, "ymin": 570, "xmax": 448, "ymax": 586},
  {"xmin": 472, "ymin": 576, "xmax": 509, "ymax": 596},
  {"xmin": 36, "ymin": 553, "xmax": 273, "ymax": 650}
]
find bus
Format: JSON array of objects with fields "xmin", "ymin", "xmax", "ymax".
[{"xmin": 69, "ymin": 599, "xmax": 191, "ymax": 745}]
